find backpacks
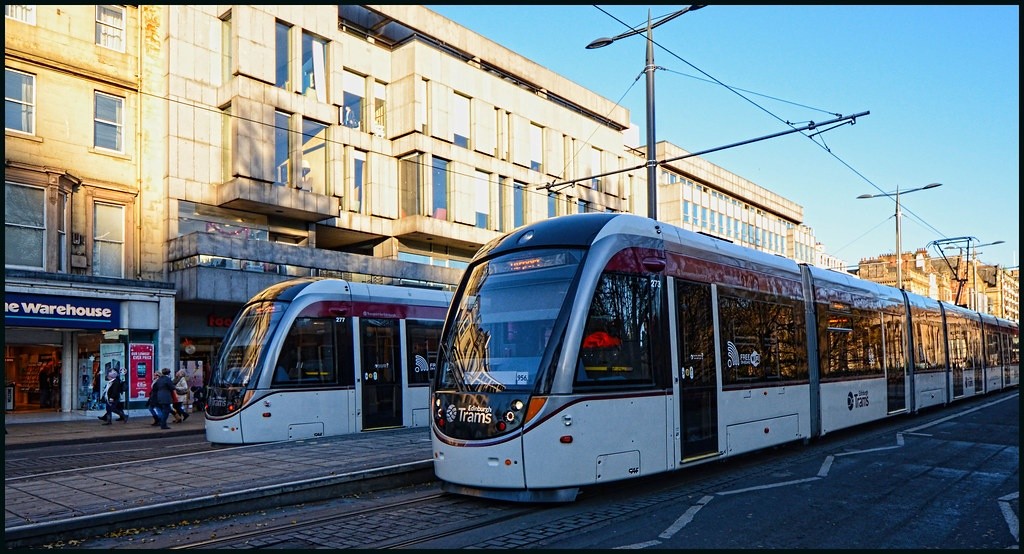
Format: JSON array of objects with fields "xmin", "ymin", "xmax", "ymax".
[{"xmin": 53, "ymin": 376, "xmax": 59, "ymax": 387}]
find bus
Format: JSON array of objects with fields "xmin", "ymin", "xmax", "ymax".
[
  {"xmin": 430, "ymin": 210, "xmax": 1017, "ymax": 505},
  {"xmin": 203, "ymin": 275, "xmax": 489, "ymax": 450}
]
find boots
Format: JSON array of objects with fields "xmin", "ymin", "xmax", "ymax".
[
  {"xmin": 175, "ymin": 415, "xmax": 182, "ymax": 423},
  {"xmin": 152, "ymin": 415, "xmax": 159, "ymax": 426}
]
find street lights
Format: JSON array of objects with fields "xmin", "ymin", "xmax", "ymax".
[
  {"xmin": 856, "ymin": 182, "xmax": 944, "ymax": 289},
  {"xmin": 942, "ymin": 240, "xmax": 1008, "ymax": 311},
  {"xmin": 586, "ymin": 3, "xmax": 706, "ymax": 220}
]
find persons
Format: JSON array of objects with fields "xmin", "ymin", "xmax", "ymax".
[
  {"xmin": 49, "ymin": 366, "xmax": 61, "ymax": 407},
  {"xmin": 203, "ymin": 362, "xmax": 211, "ymax": 386},
  {"xmin": 279, "ymin": 346, "xmax": 297, "ymax": 379},
  {"xmin": 244, "ymin": 350, "xmax": 290, "ymax": 381},
  {"xmin": 190, "ymin": 368, "xmax": 203, "ymax": 411},
  {"xmin": 148, "ymin": 368, "xmax": 189, "ymax": 430},
  {"xmin": 39, "ymin": 364, "xmax": 54, "ymax": 408},
  {"xmin": 533, "ymin": 325, "xmax": 588, "ymax": 381},
  {"xmin": 97, "ymin": 368, "xmax": 129, "ymax": 425},
  {"xmin": 361, "ymin": 332, "xmax": 391, "ymax": 380}
]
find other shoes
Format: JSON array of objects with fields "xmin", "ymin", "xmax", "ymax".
[
  {"xmin": 162, "ymin": 426, "xmax": 170, "ymax": 429},
  {"xmin": 98, "ymin": 416, "xmax": 107, "ymax": 422},
  {"xmin": 124, "ymin": 415, "xmax": 128, "ymax": 423},
  {"xmin": 183, "ymin": 413, "xmax": 189, "ymax": 421},
  {"xmin": 116, "ymin": 417, "xmax": 123, "ymax": 421},
  {"xmin": 102, "ymin": 421, "xmax": 112, "ymax": 425}
]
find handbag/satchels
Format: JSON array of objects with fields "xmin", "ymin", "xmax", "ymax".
[{"xmin": 176, "ymin": 389, "xmax": 188, "ymax": 395}]
